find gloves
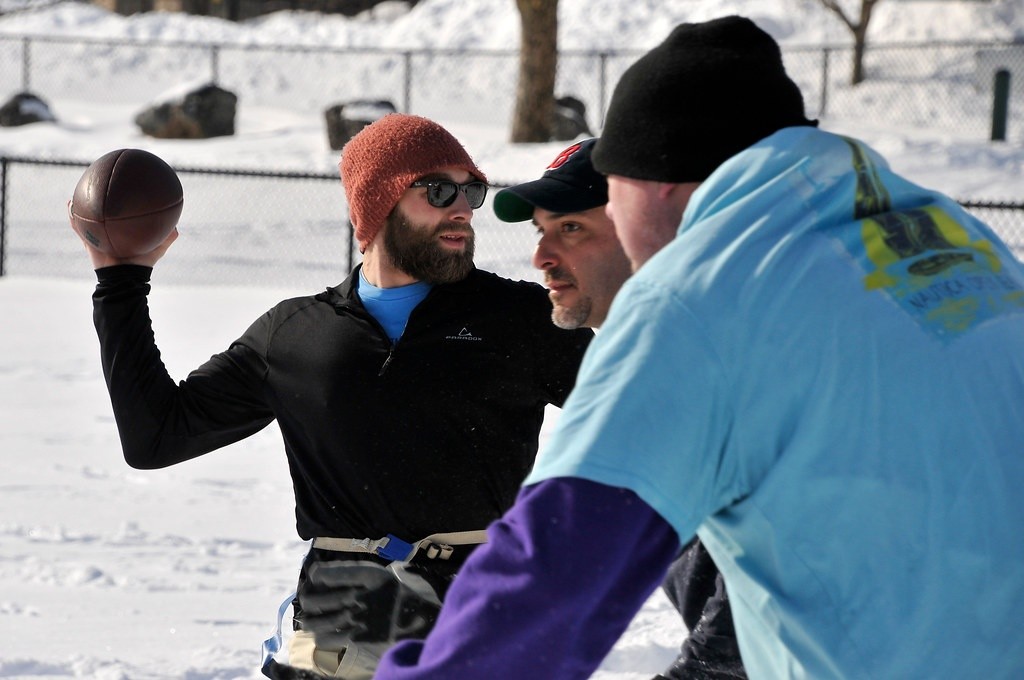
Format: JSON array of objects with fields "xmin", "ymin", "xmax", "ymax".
[{"xmin": 298, "ymin": 557, "xmax": 452, "ymax": 647}]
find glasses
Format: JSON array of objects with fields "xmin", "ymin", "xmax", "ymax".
[{"xmin": 407, "ymin": 180, "xmax": 490, "ymax": 209}]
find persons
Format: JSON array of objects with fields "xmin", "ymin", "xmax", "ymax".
[
  {"xmin": 67, "ymin": 112, "xmax": 596, "ymax": 680},
  {"xmin": 373, "ymin": 13, "xmax": 1024, "ymax": 680},
  {"xmin": 492, "ymin": 139, "xmax": 749, "ymax": 680}
]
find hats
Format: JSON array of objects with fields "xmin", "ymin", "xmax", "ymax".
[
  {"xmin": 493, "ymin": 138, "xmax": 609, "ymax": 223},
  {"xmin": 339, "ymin": 112, "xmax": 489, "ymax": 254},
  {"xmin": 590, "ymin": 16, "xmax": 819, "ymax": 184}
]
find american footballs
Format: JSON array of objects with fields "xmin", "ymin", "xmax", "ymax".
[{"xmin": 69, "ymin": 147, "xmax": 184, "ymax": 259}]
